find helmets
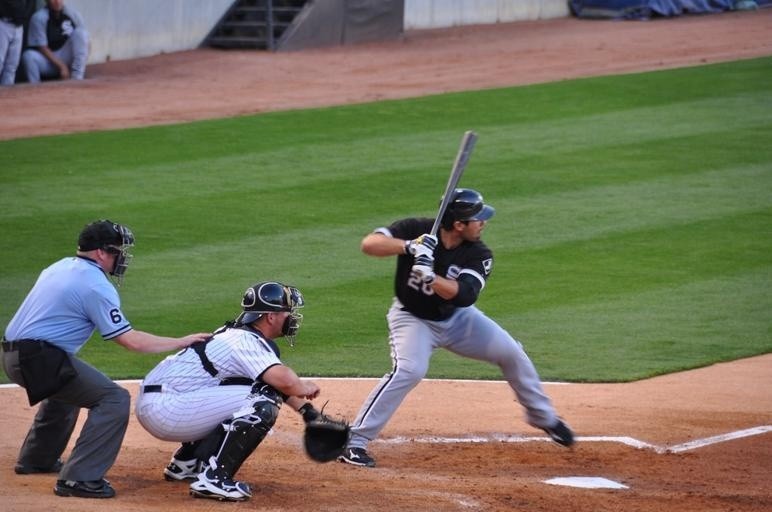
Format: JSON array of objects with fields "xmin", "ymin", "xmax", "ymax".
[
  {"xmin": 235, "ymin": 281, "xmax": 306, "ymax": 348},
  {"xmin": 438, "ymin": 188, "xmax": 495, "ymax": 222},
  {"xmin": 76, "ymin": 219, "xmax": 136, "ymax": 288}
]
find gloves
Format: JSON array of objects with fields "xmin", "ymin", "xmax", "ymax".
[
  {"xmin": 411, "ymin": 252, "xmax": 436, "ymax": 286},
  {"xmin": 405, "ymin": 233, "xmax": 439, "ymax": 256}
]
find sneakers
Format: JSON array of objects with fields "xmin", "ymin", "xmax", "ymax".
[
  {"xmin": 189, "ymin": 456, "xmax": 254, "ymax": 501},
  {"xmin": 53, "ymin": 478, "xmax": 115, "ymax": 498},
  {"xmin": 336, "ymin": 447, "xmax": 376, "ymax": 467},
  {"xmin": 14, "ymin": 456, "xmax": 65, "ymax": 474},
  {"xmin": 163, "ymin": 439, "xmax": 209, "ymax": 481},
  {"xmin": 534, "ymin": 415, "xmax": 574, "ymax": 449}
]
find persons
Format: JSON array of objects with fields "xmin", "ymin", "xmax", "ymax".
[
  {"xmin": 134, "ymin": 281, "xmax": 351, "ymax": 503},
  {"xmin": 336, "ymin": 189, "xmax": 576, "ymax": 470},
  {"xmin": 0, "ymin": 0, "xmax": 45, "ymax": 86},
  {"xmin": 20, "ymin": 0, "xmax": 90, "ymax": 84},
  {"xmin": 1, "ymin": 219, "xmax": 215, "ymax": 498}
]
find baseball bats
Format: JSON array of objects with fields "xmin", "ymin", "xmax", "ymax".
[{"xmin": 416, "ymin": 130, "xmax": 478, "ymax": 276}]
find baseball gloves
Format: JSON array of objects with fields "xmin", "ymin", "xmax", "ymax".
[{"xmin": 305, "ymin": 401, "xmax": 350, "ymax": 462}]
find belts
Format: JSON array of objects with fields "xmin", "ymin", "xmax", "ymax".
[
  {"xmin": 144, "ymin": 385, "xmax": 161, "ymax": 393},
  {"xmin": 0, "ymin": 335, "xmax": 19, "ymax": 352}
]
